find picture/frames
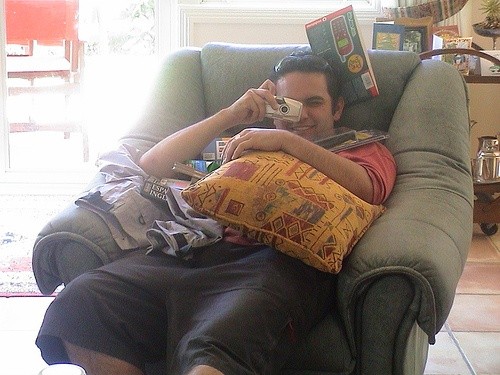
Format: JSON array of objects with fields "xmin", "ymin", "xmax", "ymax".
[{"xmin": 373, "ymin": 23, "xmax": 473, "ymax": 76}]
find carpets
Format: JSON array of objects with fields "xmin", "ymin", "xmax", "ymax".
[{"xmin": 0, "ymin": 253, "xmax": 64, "ymax": 297}]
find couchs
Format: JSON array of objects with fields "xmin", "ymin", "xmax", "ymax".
[{"xmin": 32, "ymin": 42, "xmax": 473, "ymax": 375}]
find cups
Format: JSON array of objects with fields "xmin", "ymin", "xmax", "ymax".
[{"xmin": 40, "ymin": 364, "xmax": 87, "ymax": 375}]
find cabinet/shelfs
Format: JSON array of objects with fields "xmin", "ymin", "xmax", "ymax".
[{"xmin": 473, "ymin": 181, "xmax": 500, "ymax": 225}]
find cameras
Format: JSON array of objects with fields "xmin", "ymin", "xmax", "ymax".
[{"xmin": 263, "ymin": 96, "xmax": 303, "ymax": 122}]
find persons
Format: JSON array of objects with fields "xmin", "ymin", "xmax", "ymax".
[{"xmin": 36, "ymin": 51, "xmax": 396, "ymax": 375}]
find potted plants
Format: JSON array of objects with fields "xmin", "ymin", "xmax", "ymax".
[{"xmin": 472, "ymin": 0, "xmax": 500, "ymax": 38}]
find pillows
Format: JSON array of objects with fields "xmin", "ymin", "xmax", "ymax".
[{"xmin": 180, "ymin": 146, "xmax": 385, "ymax": 274}]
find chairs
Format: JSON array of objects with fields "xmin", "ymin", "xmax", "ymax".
[{"xmin": 4, "ymin": 0, "xmax": 90, "ymax": 168}]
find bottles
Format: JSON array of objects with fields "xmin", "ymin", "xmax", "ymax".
[{"xmin": 476, "ymin": 140, "xmax": 500, "ymax": 181}]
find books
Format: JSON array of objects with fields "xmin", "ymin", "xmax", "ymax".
[
  {"xmin": 141, "ymin": 138, "xmax": 237, "ymax": 202},
  {"xmin": 305, "ymin": 4, "xmax": 380, "ymax": 107},
  {"xmin": 302, "ymin": 124, "xmax": 390, "ymax": 154}
]
